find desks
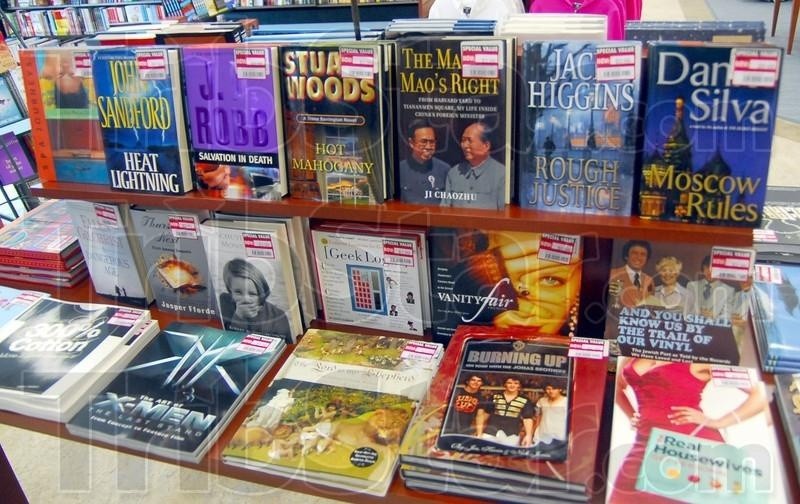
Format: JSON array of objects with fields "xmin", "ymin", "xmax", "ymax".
[{"xmin": 0, "ymin": 274, "xmax": 799, "ymax": 504}]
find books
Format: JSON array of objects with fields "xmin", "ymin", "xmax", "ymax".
[{"xmin": 0, "ymin": 0, "xmax": 799, "ymax": 504}]
